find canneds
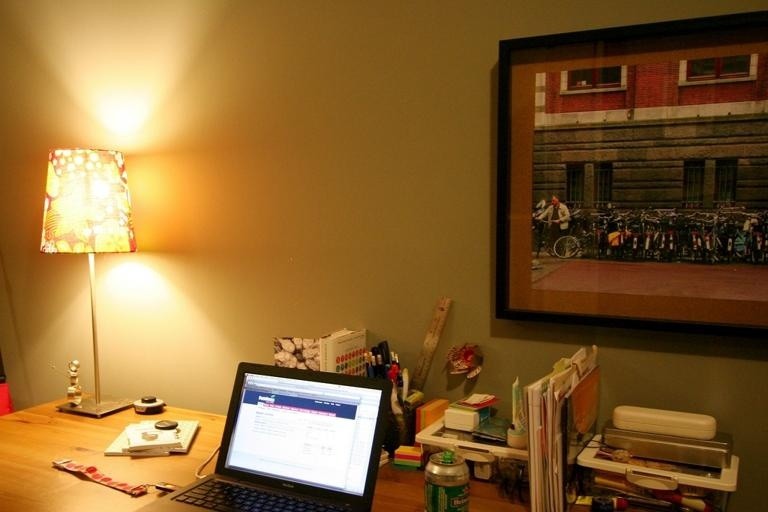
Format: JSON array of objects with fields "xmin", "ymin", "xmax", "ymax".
[{"xmin": 424, "ymin": 448, "xmax": 470, "ymax": 511}]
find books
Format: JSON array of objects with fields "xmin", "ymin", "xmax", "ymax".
[
  {"xmin": 415, "ymin": 398, "xmax": 449, "ymax": 445},
  {"xmin": 320, "ymin": 327, "xmax": 368, "ymax": 375},
  {"xmin": 525, "ymin": 345, "xmax": 598, "ymax": 512},
  {"xmin": 105, "ymin": 417, "xmax": 199, "ymax": 459}
]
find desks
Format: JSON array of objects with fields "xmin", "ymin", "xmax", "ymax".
[{"xmin": 0, "ymin": 395, "xmax": 589, "ymax": 512}]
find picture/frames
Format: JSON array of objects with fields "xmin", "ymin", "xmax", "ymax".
[{"xmin": 495, "ymin": 8, "xmax": 768, "ymax": 340}]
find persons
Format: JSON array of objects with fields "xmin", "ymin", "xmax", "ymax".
[{"xmin": 538, "ymin": 195, "xmax": 570, "ymax": 256}]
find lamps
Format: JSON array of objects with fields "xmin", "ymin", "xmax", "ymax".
[{"xmin": 38, "ymin": 147, "xmax": 137, "ymax": 418}]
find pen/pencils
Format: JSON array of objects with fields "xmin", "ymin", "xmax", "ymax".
[{"xmin": 363, "ymin": 339, "xmax": 403, "ymax": 389}]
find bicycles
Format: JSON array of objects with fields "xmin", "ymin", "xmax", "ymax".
[{"xmin": 530, "ymin": 204, "xmax": 768, "ymax": 264}]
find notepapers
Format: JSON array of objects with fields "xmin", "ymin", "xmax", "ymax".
[
  {"xmin": 394, "ymin": 445, "xmax": 423, "ymax": 471},
  {"xmin": 415, "ymin": 399, "xmax": 449, "ymax": 447}
]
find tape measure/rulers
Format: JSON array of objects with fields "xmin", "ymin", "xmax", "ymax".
[{"xmin": 408, "ymin": 295, "xmax": 453, "ymax": 392}]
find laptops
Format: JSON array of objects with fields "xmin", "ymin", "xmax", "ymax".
[{"xmin": 133, "ymin": 362, "xmax": 393, "ymax": 512}]
